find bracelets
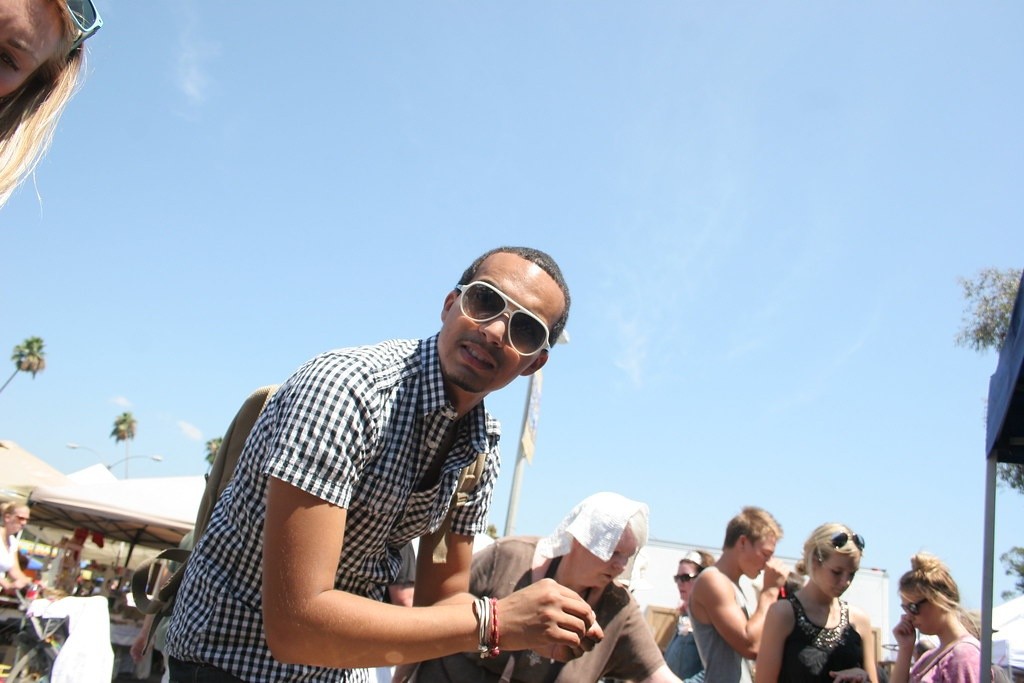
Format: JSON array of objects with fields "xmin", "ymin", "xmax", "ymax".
[{"xmin": 474, "ymin": 596, "xmax": 499, "ymax": 660}]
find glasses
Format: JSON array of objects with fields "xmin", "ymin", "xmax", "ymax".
[
  {"xmin": 64, "ymin": 0, "xmax": 102, "ymax": 52},
  {"xmin": 900, "ymin": 597, "xmax": 928, "ymax": 615},
  {"xmin": 830, "ymin": 531, "xmax": 866, "ymax": 551},
  {"xmin": 673, "ymin": 573, "xmax": 697, "ymax": 583},
  {"xmin": 457, "ymin": 280, "xmax": 550, "ymax": 357},
  {"xmin": 15, "ymin": 515, "xmax": 29, "ymax": 524}
]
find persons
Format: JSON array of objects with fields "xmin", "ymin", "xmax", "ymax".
[
  {"xmin": 890, "ymin": 553, "xmax": 1012, "ymax": 683},
  {"xmin": 132, "ymin": 246, "xmax": 604, "ymax": 683},
  {"xmin": 394, "ymin": 492, "xmax": 688, "ymax": 683},
  {"xmin": 0, "ymin": 502, "xmax": 34, "ymax": 592},
  {"xmin": 658, "ymin": 506, "xmax": 790, "ymax": 683},
  {"xmin": 0, "ymin": 0, "xmax": 103, "ymax": 207},
  {"xmin": 755, "ymin": 522, "xmax": 880, "ymax": 683}
]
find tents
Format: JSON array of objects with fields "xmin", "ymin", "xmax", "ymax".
[
  {"xmin": 1, "ymin": 440, "xmax": 212, "ymax": 573},
  {"xmin": 916, "ymin": 269, "xmax": 1024, "ymax": 683}
]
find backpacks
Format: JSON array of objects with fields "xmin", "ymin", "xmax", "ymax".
[{"xmin": 129, "ymin": 386, "xmax": 278, "ymax": 662}]
